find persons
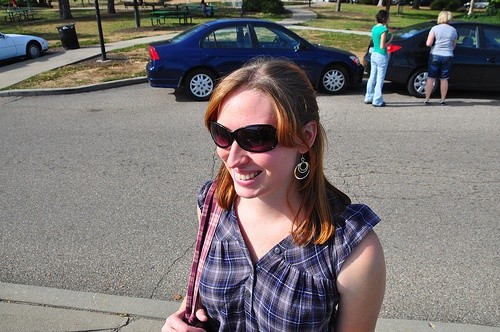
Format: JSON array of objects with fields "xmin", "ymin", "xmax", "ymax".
[
  {"xmin": 201, "ymin": 0, "xmax": 208, "ymax": 11},
  {"xmin": 12, "ymin": 0, "xmax": 16, "ymax": 7},
  {"xmin": 424, "ymin": 10, "xmax": 458, "ymax": 105},
  {"xmin": 162, "ymin": 60, "xmax": 386, "ymax": 332},
  {"xmin": 364, "ymin": 10, "xmax": 393, "ymax": 107}
]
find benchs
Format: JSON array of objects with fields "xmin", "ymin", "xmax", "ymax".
[
  {"xmin": 4, "ymin": 13, "xmax": 37, "ymax": 22},
  {"xmin": 151, "ymin": 15, "xmax": 193, "ymax": 25}
]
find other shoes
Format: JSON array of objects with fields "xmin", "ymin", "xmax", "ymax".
[
  {"xmin": 425, "ymin": 100, "xmax": 431, "ymax": 106},
  {"xmin": 365, "ymin": 101, "xmax": 372, "ymax": 104},
  {"xmin": 439, "ymin": 100, "xmax": 445, "ymax": 106},
  {"xmin": 372, "ymin": 101, "xmax": 386, "ymax": 107}
]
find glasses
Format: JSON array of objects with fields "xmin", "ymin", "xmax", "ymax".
[{"xmin": 210, "ymin": 120, "xmax": 278, "ymax": 152}]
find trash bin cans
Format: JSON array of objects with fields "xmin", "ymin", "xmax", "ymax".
[{"xmin": 56, "ymin": 23, "xmax": 79, "ymax": 49}]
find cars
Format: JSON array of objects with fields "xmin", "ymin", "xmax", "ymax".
[
  {"xmin": 362, "ymin": 21, "xmax": 500, "ymax": 98},
  {"xmin": 145, "ymin": 17, "xmax": 365, "ymax": 100},
  {"xmin": 0, "ymin": 32, "xmax": 50, "ymax": 61},
  {"xmin": 327, "ymin": 0, "xmax": 490, "ymax": 10}
]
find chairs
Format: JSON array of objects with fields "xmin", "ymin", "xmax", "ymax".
[
  {"xmin": 243, "ymin": 33, "xmax": 251, "ymax": 47},
  {"xmin": 463, "ymin": 36, "xmax": 473, "ymax": 46}
]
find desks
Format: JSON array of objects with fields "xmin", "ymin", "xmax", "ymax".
[
  {"xmin": 151, "ymin": 10, "xmax": 190, "ymax": 25},
  {"xmin": 4, "ymin": 10, "xmax": 29, "ymax": 22}
]
